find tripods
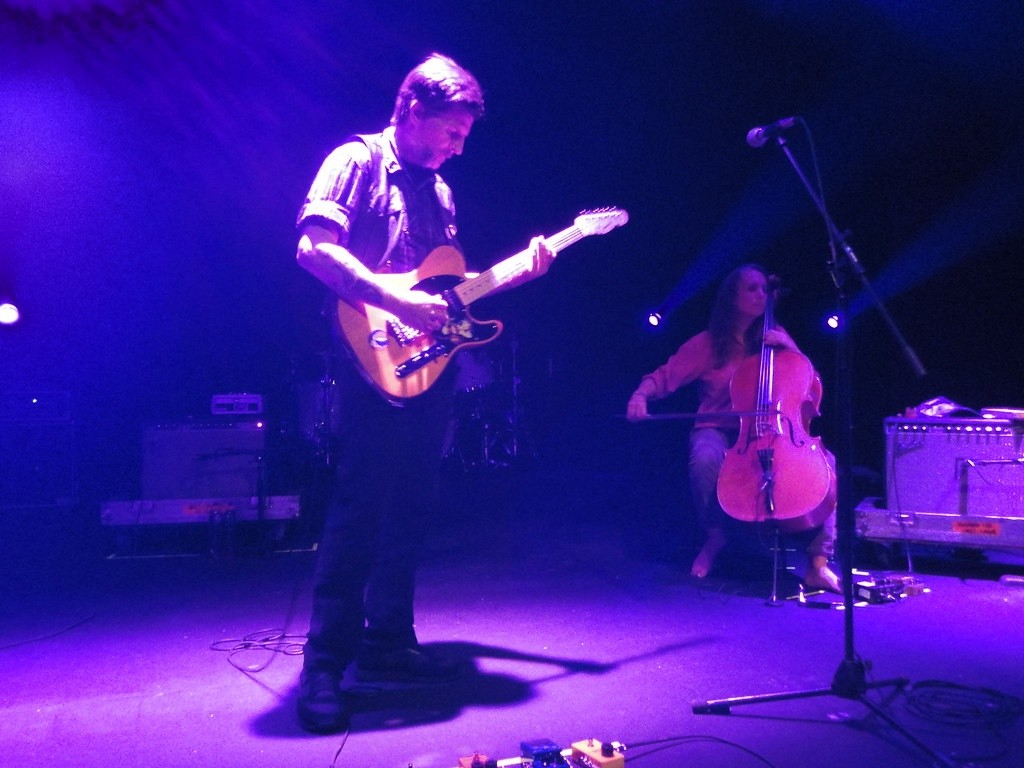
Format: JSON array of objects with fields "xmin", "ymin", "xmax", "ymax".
[{"xmin": 691, "ymin": 138, "xmax": 960, "ymax": 768}]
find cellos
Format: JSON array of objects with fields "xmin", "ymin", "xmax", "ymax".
[{"xmin": 715, "ymin": 270, "xmax": 837, "ymax": 610}]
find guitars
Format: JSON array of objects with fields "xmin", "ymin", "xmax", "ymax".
[{"xmin": 332, "ymin": 206, "xmax": 630, "ymax": 409}]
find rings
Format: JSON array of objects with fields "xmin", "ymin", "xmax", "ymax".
[{"xmin": 430, "ymin": 311, "xmax": 434, "ymax": 315}]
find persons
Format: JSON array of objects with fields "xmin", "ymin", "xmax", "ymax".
[
  {"xmin": 293, "ymin": 51, "xmax": 558, "ymax": 734},
  {"xmin": 627, "ymin": 265, "xmax": 841, "ymax": 591}
]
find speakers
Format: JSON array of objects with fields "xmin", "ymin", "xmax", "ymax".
[
  {"xmin": 884, "ymin": 417, "xmax": 1023, "ymax": 518},
  {"xmin": 139, "ymin": 417, "xmax": 265, "ymax": 499}
]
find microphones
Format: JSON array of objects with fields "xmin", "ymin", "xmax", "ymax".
[{"xmin": 746, "ymin": 113, "xmax": 804, "ymax": 149}]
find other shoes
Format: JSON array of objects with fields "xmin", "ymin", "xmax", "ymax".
[
  {"xmin": 355, "ymin": 645, "xmax": 473, "ymax": 690},
  {"xmin": 298, "ymin": 658, "xmax": 350, "ymax": 732}
]
